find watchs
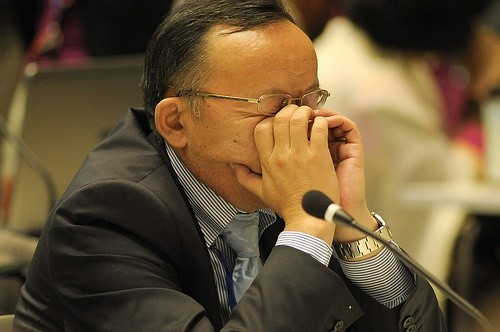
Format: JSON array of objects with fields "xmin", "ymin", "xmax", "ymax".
[{"xmin": 332, "ymin": 211, "xmax": 394, "ymax": 259}]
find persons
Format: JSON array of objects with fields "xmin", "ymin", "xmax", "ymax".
[{"xmin": 14, "ymin": 1, "xmax": 500, "ymax": 332}]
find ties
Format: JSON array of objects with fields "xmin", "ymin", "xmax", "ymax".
[{"xmin": 218, "ymin": 211, "xmax": 264, "ymax": 304}]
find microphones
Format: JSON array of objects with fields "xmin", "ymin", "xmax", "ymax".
[{"xmin": 301, "ymin": 190, "xmax": 496, "ymax": 332}]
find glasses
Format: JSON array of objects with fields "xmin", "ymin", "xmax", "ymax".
[{"xmin": 176, "ymin": 89, "xmax": 331, "ymax": 116}]
